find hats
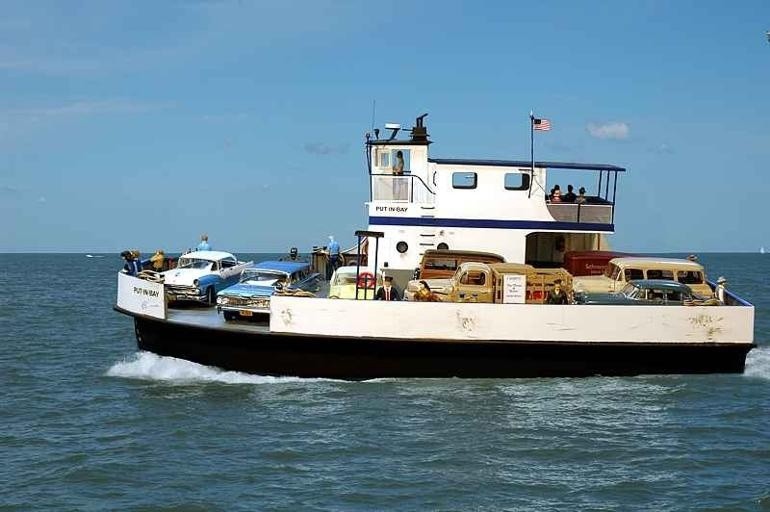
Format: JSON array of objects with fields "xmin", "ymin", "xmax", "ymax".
[
  {"xmin": 553, "ymin": 279, "xmax": 562, "ymax": 284},
  {"xmin": 384, "ymin": 276, "xmax": 393, "ymax": 281},
  {"xmin": 716, "ymin": 276, "xmax": 727, "ymax": 283}
]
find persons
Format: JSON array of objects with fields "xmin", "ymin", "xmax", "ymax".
[
  {"xmin": 685, "ymin": 255, "xmax": 699, "ymax": 279},
  {"xmin": 546, "ymin": 278, "xmax": 568, "ymax": 304},
  {"xmin": 131, "ymin": 250, "xmax": 144, "ymax": 276},
  {"xmin": 413, "ymin": 281, "xmax": 440, "ymax": 302},
  {"xmin": 149, "ymin": 249, "xmax": 164, "ymax": 277},
  {"xmin": 196, "ymin": 234, "xmax": 211, "ymax": 262},
  {"xmin": 374, "ymin": 276, "xmax": 401, "ymax": 301},
  {"xmin": 120, "ymin": 250, "xmax": 136, "ymax": 277},
  {"xmin": 326, "ymin": 236, "xmax": 341, "ymax": 280},
  {"xmin": 715, "ymin": 276, "xmax": 728, "ymax": 305},
  {"xmin": 392, "ymin": 151, "xmax": 405, "ymax": 200},
  {"xmin": 549, "ymin": 183, "xmax": 588, "ymax": 204}
]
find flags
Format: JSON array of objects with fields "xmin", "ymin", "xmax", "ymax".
[{"xmin": 532, "ymin": 119, "xmax": 551, "ymax": 130}]
